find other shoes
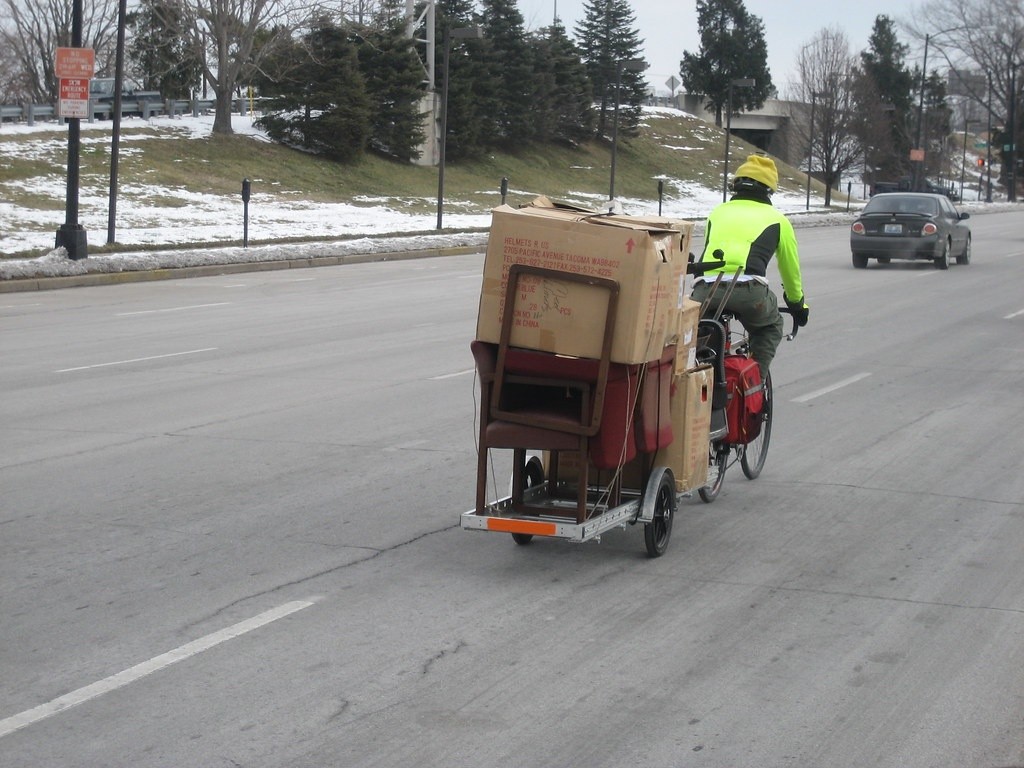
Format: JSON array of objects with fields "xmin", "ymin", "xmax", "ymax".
[{"xmin": 760, "ymin": 401, "xmax": 769, "ymax": 413}]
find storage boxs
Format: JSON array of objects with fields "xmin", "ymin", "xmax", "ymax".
[
  {"xmin": 476, "ymin": 198, "xmax": 695, "ymax": 362},
  {"xmin": 540, "ymin": 297, "xmax": 714, "ymax": 494}
]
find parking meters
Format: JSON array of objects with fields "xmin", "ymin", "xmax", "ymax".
[
  {"xmin": 500, "ymin": 177, "xmax": 508, "ymax": 205},
  {"xmin": 240, "ymin": 177, "xmax": 251, "ymax": 251},
  {"xmin": 657, "ymin": 179, "xmax": 663, "ymax": 215},
  {"xmin": 845, "ymin": 179, "xmax": 853, "ymax": 211}
]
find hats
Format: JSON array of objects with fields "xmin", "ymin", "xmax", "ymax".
[{"xmin": 734, "ymin": 154, "xmax": 778, "ymax": 191}]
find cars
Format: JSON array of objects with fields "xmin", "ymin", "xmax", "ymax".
[{"xmin": 849, "ymin": 193, "xmax": 975, "ymax": 271}]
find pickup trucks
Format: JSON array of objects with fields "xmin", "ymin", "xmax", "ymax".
[{"xmin": 90, "ymin": 77, "xmax": 161, "ymax": 119}]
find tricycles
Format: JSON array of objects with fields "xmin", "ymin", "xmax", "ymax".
[{"xmin": 463, "ymin": 304, "xmax": 802, "ymax": 558}]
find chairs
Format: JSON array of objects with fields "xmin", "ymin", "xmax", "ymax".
[{"xmin": 470, "ymin": 264, "xmax": 674, "ymax": 524}]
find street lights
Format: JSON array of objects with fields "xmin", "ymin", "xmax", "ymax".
[
  {"xmin": 722, "ymin": 76, "xmax": 757, "ymax": 204},
  {"xmin": 608, "ymin": 58, "xmax": 649, "ymax": 217},
  {"xmin": 433, "ymin": 24, "xmax": 485, "ymax": 230},
  {"xmin": 806, "ymin": 88, "xmax": 835, "ymax": 211},
  {"xmin": 958, "ymin": 119, "xmax": 981, "ymax": 204},
  {"xmin": 910, "ymin": 24, "xmax": 981, "ymax": 192}
]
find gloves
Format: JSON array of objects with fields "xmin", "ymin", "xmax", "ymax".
[{"xmin": 783, "ymin": 291, "xmax": 809, "ymax": 327}]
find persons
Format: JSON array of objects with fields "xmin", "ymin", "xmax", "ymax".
[{"xmin": 688, "ymin": 153, "xmax": 809, "ymax": 411}]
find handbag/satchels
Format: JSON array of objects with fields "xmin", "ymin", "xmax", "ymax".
[{"xmin": 721, "ymin": 355, "xmax": 764, "ymax": 445}]
